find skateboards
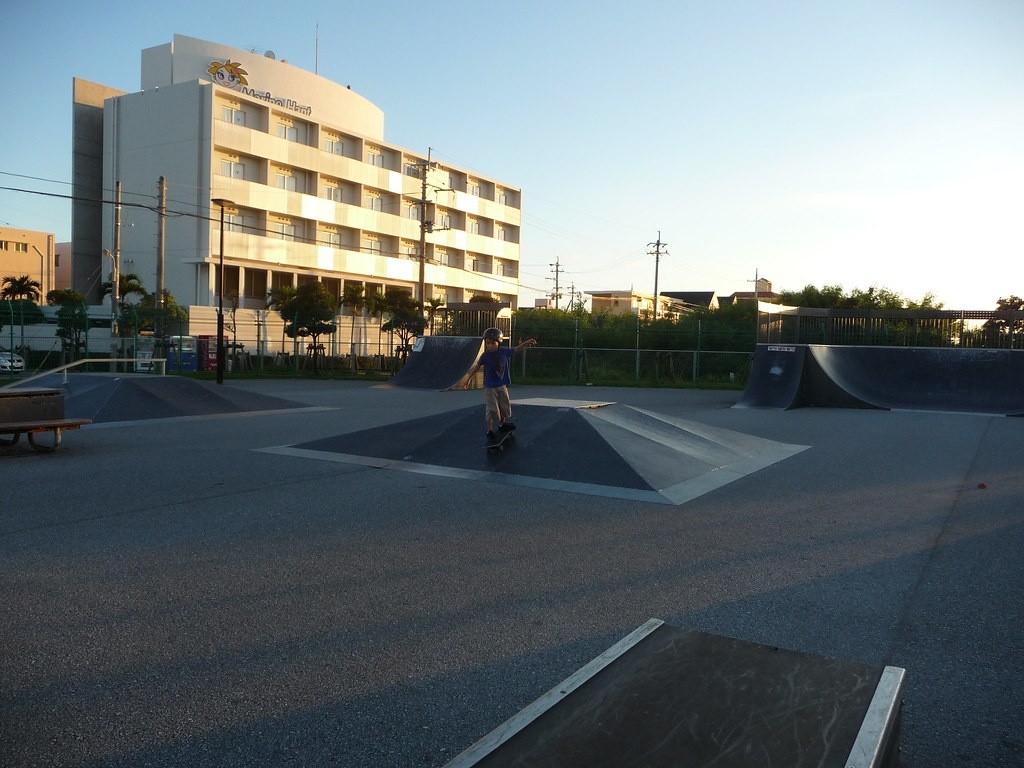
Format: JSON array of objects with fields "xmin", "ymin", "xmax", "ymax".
[{"xmin": 482, "ymin": 422, "xmax": 517, "ymax": 453}]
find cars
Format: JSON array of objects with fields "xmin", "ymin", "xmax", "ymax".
[{"xmin": 0, "ymin": 346, "xmax": 26, "ymax": 375}]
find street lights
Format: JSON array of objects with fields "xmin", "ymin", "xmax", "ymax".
[
  {"xmin": 211, "ymin": 198, "xmax": 231, "ymax": 383},
  {"xmin": 103, "ymin": 249, "xmax": 118, "ymax": 373}
]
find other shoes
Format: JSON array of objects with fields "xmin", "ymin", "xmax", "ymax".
[
  {"xmin": 486, "ymin": 430, "xmax": 499, "ymax": 443},
  {"xmin": 497, "ymin": 423, "xmax": 516, "ymax": 431}
]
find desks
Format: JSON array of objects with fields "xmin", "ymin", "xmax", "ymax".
[{"xmin": 0, "ymin": 387, "xmax": 61, "ymax": 453}]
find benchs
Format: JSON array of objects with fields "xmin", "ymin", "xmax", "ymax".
[{"xmin": 0, "ymin": 396, "xmax": 92, "ymax": 431}]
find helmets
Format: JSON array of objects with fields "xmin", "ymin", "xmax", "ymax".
[{"xmin": 482, "ymin": 328, "xmax": 503, "ymax": 343}]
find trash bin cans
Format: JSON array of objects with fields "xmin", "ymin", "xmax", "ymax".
[{"xmin": 15, "ymin": 345, "xmax": 30, "ymax": 367}]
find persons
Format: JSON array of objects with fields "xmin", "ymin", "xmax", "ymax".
[{"xmin": 462, "ymin": 327, "xmax": 537, "ymax": 440}]
